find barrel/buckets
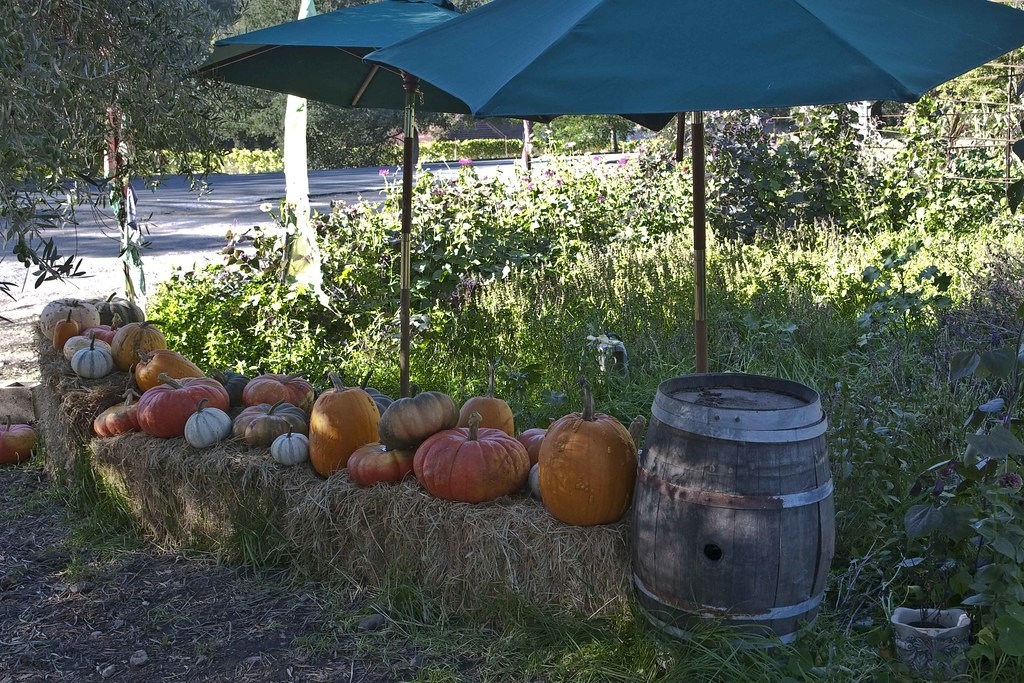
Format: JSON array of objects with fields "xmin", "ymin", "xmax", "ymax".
[{"xmin": 632, "ymin": 372, "xmax": 836, "ymax": 649}]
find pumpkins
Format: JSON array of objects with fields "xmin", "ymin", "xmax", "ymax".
[{"xmin": 0, "ymin": 414, "xmax": 38, "ymax": 463}]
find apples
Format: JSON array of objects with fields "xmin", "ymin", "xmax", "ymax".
[{"xmin": 41, "ymin": 293, "xmax": 644, "ymax": 526}]
